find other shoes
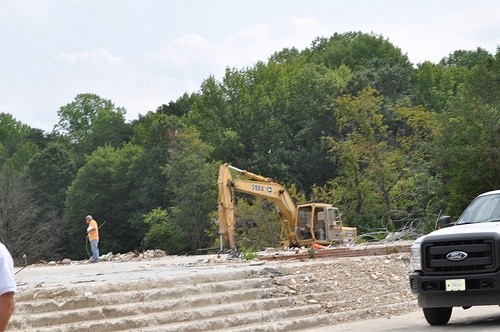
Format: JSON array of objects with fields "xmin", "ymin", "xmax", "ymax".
[{"xmin": 90, "ymin": 259, "xmax": 98, "ymax": 262}]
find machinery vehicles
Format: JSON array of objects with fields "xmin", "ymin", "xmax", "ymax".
[{"xmin": 217, "ymin": 163, "xmax": 358, "ymax": 256}]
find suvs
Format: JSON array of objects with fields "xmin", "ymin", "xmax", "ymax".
[{"xmin": 408, "ymin": 189, "xmax": 500, "ymax": 326}]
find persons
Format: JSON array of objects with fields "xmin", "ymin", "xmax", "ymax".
[
  {"xmin": 0, "ymin": 242, "xmax": 16, "ymax": 332},
  {"xmin": 85, "ymin": 215, "xmax": 100, "ymax": 263}
]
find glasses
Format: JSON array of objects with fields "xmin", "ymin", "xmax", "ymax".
[{"xmin": 86, "ymin": 218, "xmax": 90, "ymax": 220}]
